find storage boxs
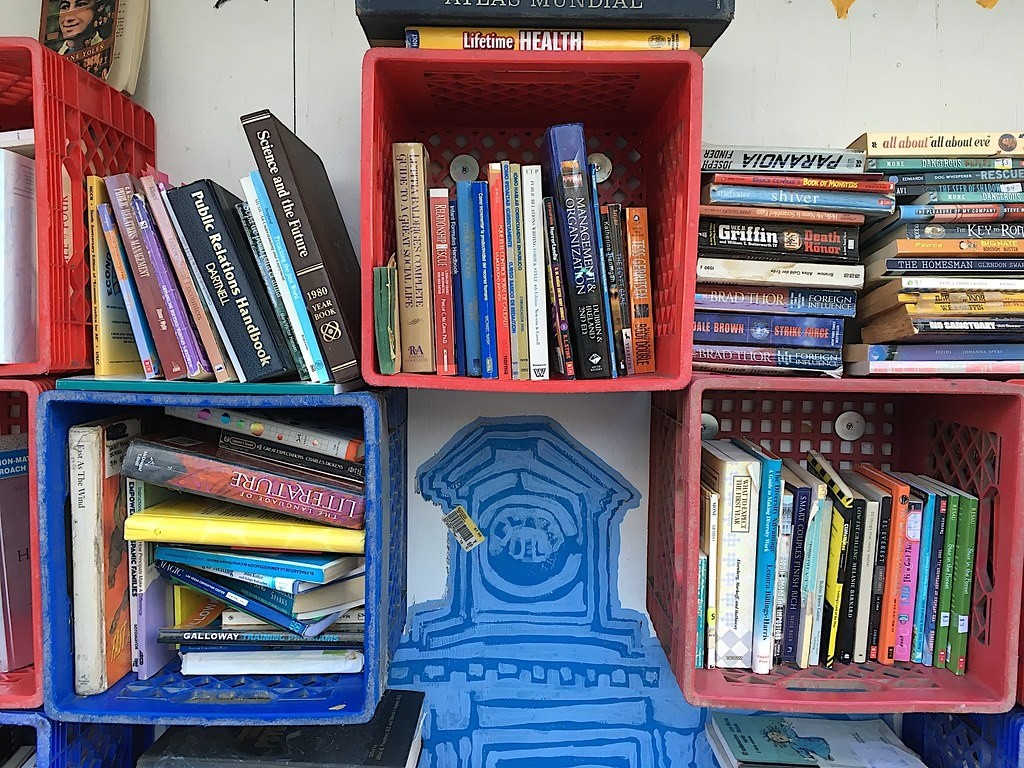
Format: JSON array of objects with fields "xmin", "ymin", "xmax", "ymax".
[{"xmin": 1, "ymin": 38, "xmax": 1024, "ymax": 768}]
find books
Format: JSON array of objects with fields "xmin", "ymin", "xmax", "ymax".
[
  {"xmin": 86, "ymin": 108, "xmax": 363, "ymax": 384},
  {"xmin": 696, "ymin": 435, "xmax": 980, "ymax": 678},
  {"xmin": 0, "ymin": 128, "xmax": 118, "ymax": 365},
  {"xmin": 55, "ymin": 372, "xmax": 367, "ymax": 396},
  {"xmin": 67, "ymin": 404, "xmax": 368, "ymax": 699},
  {"xmin": 705, "ymin": 712, "xmax": 928, "ymax": 768},
  {"xmin": 371, "ymin": 121, "xmax": 657, "ymax": 382},
  {"xmin": 690, "ymin": 131, "xmax": 1024, "ymax": 378},
  {"xmin": 354, "ymin": 1, "xmax": 735, "ymax": 59},
  {"xmin": 0, "ymin": 388, "xmax": 34, "ymax": 673}
]
